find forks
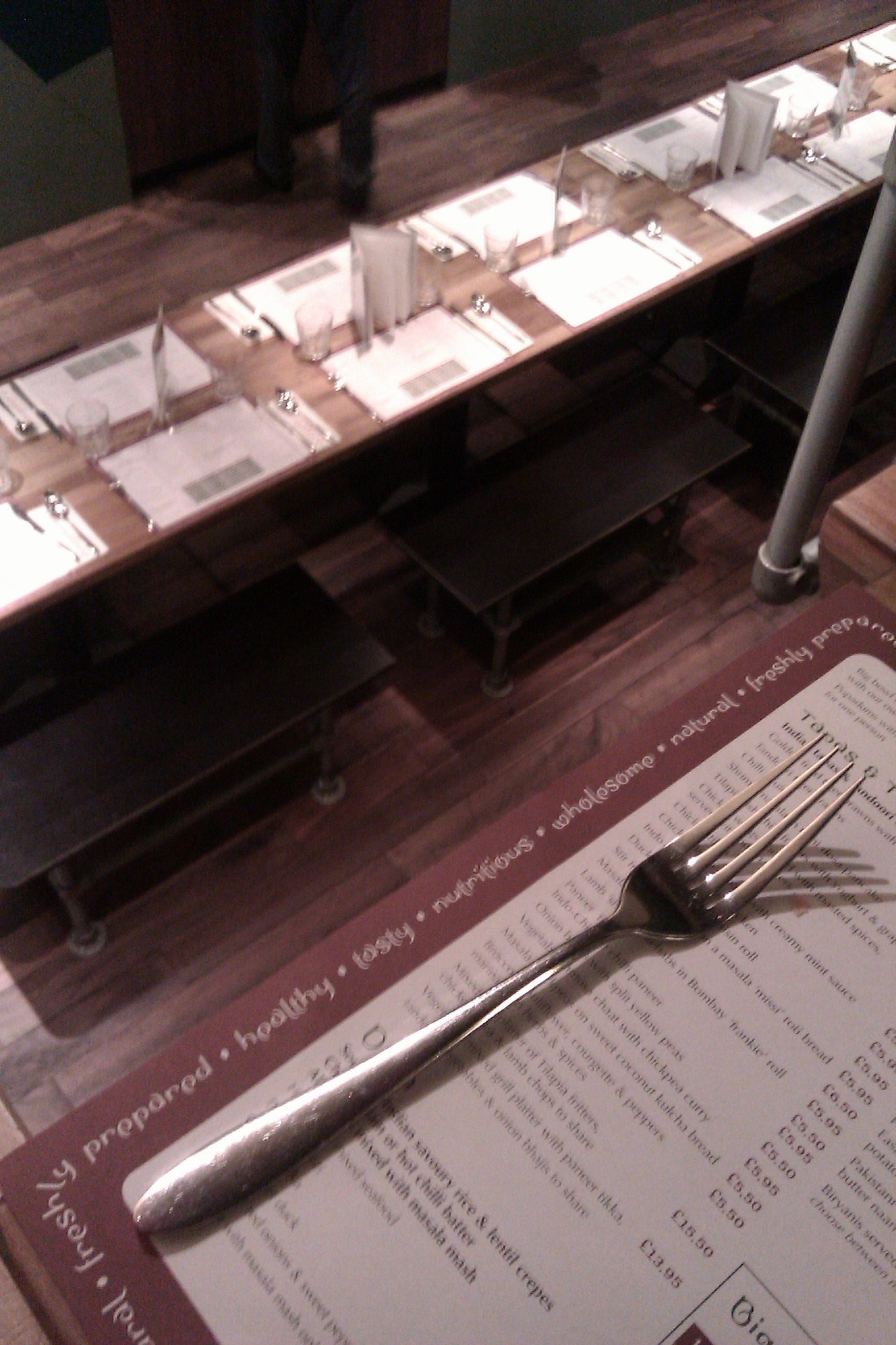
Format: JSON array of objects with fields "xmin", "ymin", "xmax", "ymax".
[{"xmin": 130, "ymin": 727, "xmax": 867, "ymax": 1236}]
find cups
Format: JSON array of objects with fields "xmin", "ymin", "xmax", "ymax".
[
  {"xmin": 0, "ymin": 439, "xmax": 12, "ymax": 495},
  {"xmin": 412, "ymin": 252, "xmax": 440, "ymax": 306},
  {"xmin": 581, "ymin": 176, "xmax": 616, "ymax": 225},
  {"xmin": 205, "ymin": 340, "xmax": 245, "ymax": 398},
  {"xmin": 847, "ymin": 64, "xmax": 876, "ymax": 110},
  {"xmin": 667, "ymin": 142, "xmax": 701, "ymax": 191},
  {"xmin": 65, "ymin": 399, "xmax": 112, "ymax": 459},
  {"xmin": 485, "ymin": 226, "xmax": 518, "ymax": 272},
  {"xmin": 294, "ymin": 302, "xmax": 335, "ymax": 360},
  {"xmin": 786, "ymin": 91, "xmax": 820, "ymax": 138}
]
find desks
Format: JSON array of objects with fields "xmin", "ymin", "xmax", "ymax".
[{"xmin": 0, "ymin": 16, "xmax": 896, "ymax": 630}]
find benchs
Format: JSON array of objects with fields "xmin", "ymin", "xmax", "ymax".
[
  {"xmin": 0, "ymin": 567, "xmax": 399, "ymax": 951},
  {"xmin": 381, "ymin": 369, "xmax": 763, "ymax": 695},
  {"xmin": 691, "ymin": 249, "xmax": 896, "ymax": 473}
]
